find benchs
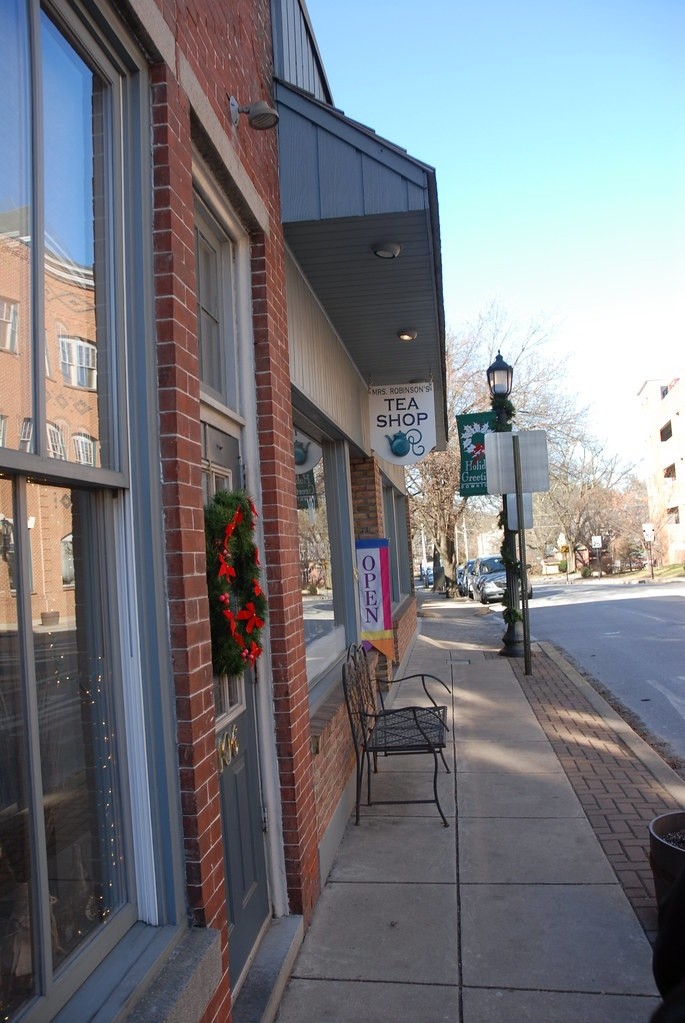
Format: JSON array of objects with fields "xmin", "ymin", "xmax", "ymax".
[{"xmin": 343, "ymin": 642, "xmax": 453, "ymax": 829}]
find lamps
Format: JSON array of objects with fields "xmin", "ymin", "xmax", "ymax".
[
  {"xmin": 229, "ymin": 95, "xmax": 281, "ymax": 130},
  {"xmin": 374, "ymin": 242, "xmax": 400, "ymax": 258},
  {"xmin": 398, "ymin": 328, "xmax": 418, "ymax": 340}
]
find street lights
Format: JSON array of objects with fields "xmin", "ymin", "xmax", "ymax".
[{"xmin": 486, "ymin": 349, "xmax": 534, "ymax": 657}]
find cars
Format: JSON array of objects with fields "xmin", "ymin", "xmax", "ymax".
[
  {"xmin": 456, "ymin": 559, "xmax": 476, "ymax": 599},
  {"xmin": 420, "ymin": 569, "xmax": 425, "ymax": 577},
  {"xmin": 426, "ymin": 570, "xmax": 433, "ymax": 585},
  {"xmin": 471, "ymin": 556, "xmax": 534, "ymax": 603}
]
